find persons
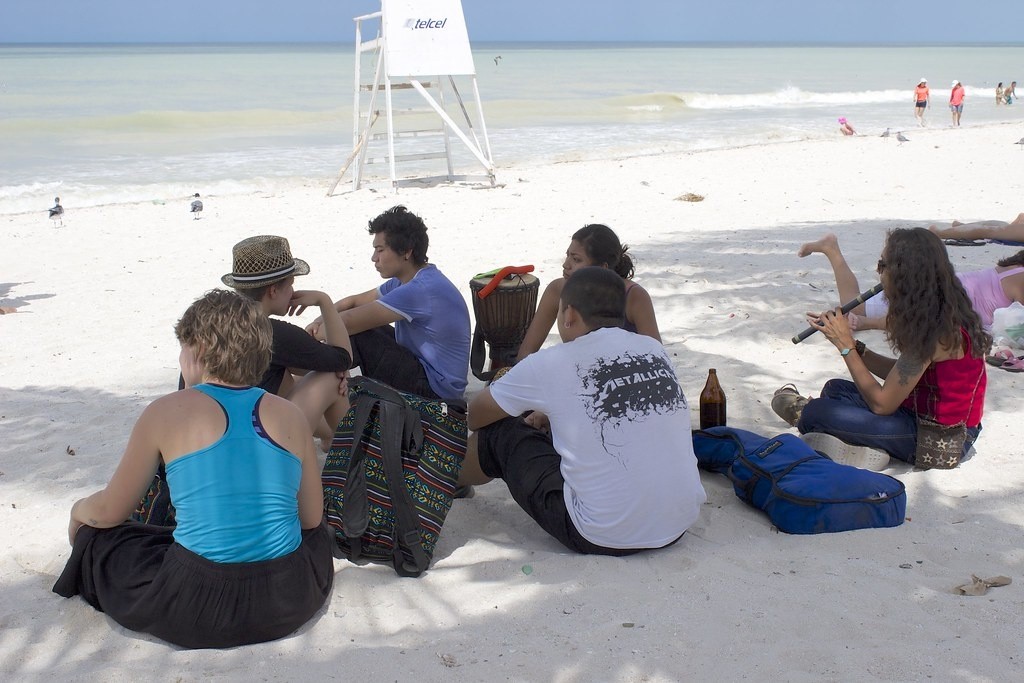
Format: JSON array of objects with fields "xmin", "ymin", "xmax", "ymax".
[
  {"xmin": 914, "ymin": 77, "xmax": 931, "ymax": 126},
  {"xmin": 514, "ymin": 224, "xmax": 663, "ymax": 364},
  {"xmin": 455, "ymin": 266, "xmax": 707, "ymax": 557},
  {"xmin": 839, "ymin": 116, "xmax": 855, "ymax": 135},
  {"xmin": 949, "ymin": 79, "xmax": 965, "ymax": 127},
  {"xmin": 772, "ymin": 227, "xmax": 986, "ymax": 472},
  {"xmin": 995, "ymin": 81, "xmax": 1018, "ymax": 105},
  {"xmin": 218, "ymin": 234, "xmax": 351, "ymax": 452},
  {"xmin": 53, "ymin": 289, "xmax": 333, "ymax": 652},
  {"xmin": 304, "ymin": 205, "xmax": 471, "ymax": 402},
  {"xmin": 927, "ymin": 212, "xmax": 1024, "ymax": 242},
  {"xmin": 797, "ymin": 233, "xmax": 1024, "ymax": 331}
]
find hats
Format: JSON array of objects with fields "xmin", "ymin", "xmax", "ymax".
[
  {"xmin": 838, "ymin": 116, "xmax": 847, "ymax": 124},
  {"xmin": 951, "ymin": 80, "xmax": 959, "ymax": 88},
  {"xmin": 917, "ymin": 77, "xmax": 927, "ymax": 85},
  {"xmin": 221, "ymin": 235, "xmax": 310, "ymax": 289}
]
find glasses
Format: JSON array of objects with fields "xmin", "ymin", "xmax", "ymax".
[{"xmin": 878, "ymin": 260, "xmax": 901, "ymax": 274}]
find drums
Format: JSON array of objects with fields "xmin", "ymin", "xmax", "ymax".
[{"xmin": 470, "ymin": 269, "xmax": 541, "ymax": 390}]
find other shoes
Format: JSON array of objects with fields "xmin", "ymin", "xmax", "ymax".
[
  {"xmin": 944, "ymin": 237, "xmax": 986, "ymax": 246},
  {"xmin": 771, "ymin": 383, "xmax": 809, "ymax": 427}
]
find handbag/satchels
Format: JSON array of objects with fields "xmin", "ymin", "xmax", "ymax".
[{"xmin": 692, "ymin": 426, "xmax": 907, "ymax": 534}]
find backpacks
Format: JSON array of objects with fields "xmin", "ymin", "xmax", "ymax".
[{"xmin": 320, "ymin": 375, "xmax": 467, "ymax": 578}]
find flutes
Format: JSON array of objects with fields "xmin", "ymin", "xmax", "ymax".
[{"xmin": 791, "ymin": 280, "xmax": 885, "ymax": 344}]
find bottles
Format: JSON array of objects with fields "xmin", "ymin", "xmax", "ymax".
[{"xmin": 700, "ymin": 368, "xmax": 726, "ymax": 430}]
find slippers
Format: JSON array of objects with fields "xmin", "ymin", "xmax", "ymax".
[{"xmin": 985, "ymin": 349, "xmax": 1024, "ymax": 372}]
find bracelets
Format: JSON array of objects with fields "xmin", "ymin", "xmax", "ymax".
[
  {"xmin": 855, "ymin": 338, "xmax": 865, "ymax": 356},
  {"xmin": 841, "ymin": 346, "xmax": 856, "ymax": 356}
]
